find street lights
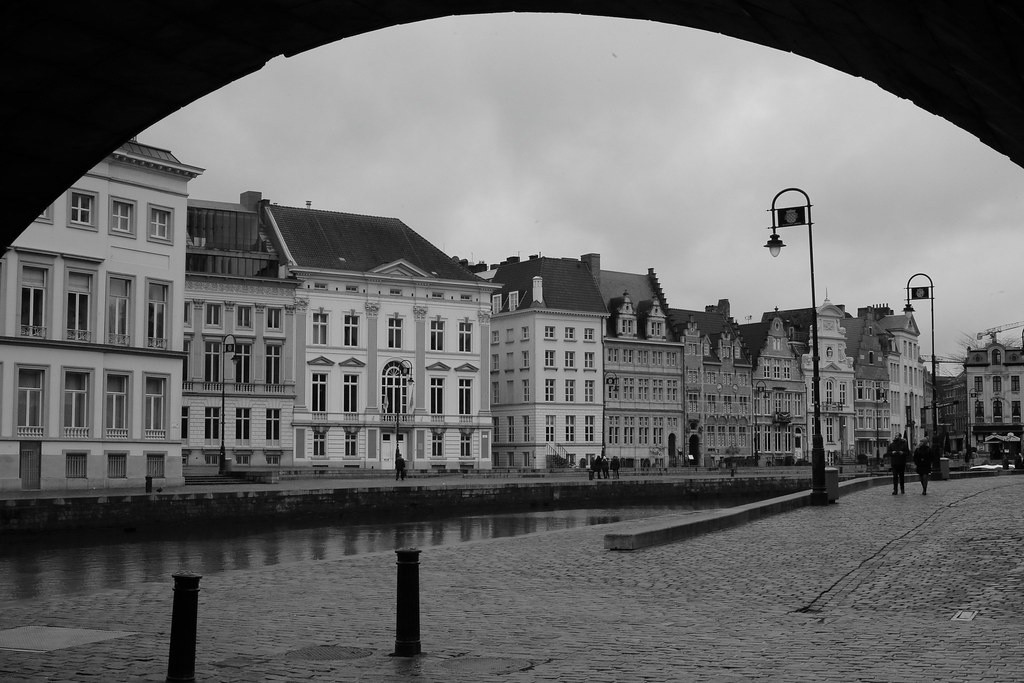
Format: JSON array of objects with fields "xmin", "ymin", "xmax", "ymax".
[
  {"xmin": 601, "ymin": 371, "xmax": 620, "ymax": 458},
  {"xmin": 762, "ymin": 185, "xmax": 829, "ymax": 506},
  {"xmin": 394, "ymin": 359, "xmax": 415, "ymax": 470},
  {"xmin": 754, "ymin": 379, "xmax": 770, "ymax": 464},
  {"xmin": 902, "ymin": 273, "xmax": 940, "ymax": 435},
  {"xmin": 217, "ymin": 332, "xmax": 243, "ymax": 477},
  {"xmin": 875, "ymin": 386, "xmax": 890, "ymax": 459}
]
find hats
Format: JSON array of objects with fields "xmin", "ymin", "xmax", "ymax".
[{"xmin": 920, "ymin": 436, "xmax": 929, "ymax": 443}]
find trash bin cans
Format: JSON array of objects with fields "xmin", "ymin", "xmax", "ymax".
[
  {"xmin": 939, "ymin": 457, "xmax": 950, "ymax": 481},
  {"xmin": 730, "ymin": 468, "xmax": 735, "ymax": 477},
  {"xmin": 825, "ymin": 466, "xmax": 839, "ymax": 504},
  {"xmin": 588, "ymin": 470, "xmax": 594, "ymax": 480},
  {"xmin": 145, "ymin": 474, "xmax": 153, "ymax": 493}
]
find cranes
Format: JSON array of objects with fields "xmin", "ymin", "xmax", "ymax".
[
  {"xmin": 919, "ymin": 353, "xmax": 968, "ymax": 364},
  {"xmin": 976, "ymin": 320, "xmax": 1024, "ymax": 343}
]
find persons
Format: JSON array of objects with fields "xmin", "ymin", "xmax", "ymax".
[
  {"xmin": 914, "ymin": 436, "xmax": 933, "ymax": 496},
  {"xmin": 590, "ymin": 454, "xmax": 620, "ymax": 479},
  {"xmin": 395, "ymin": 454, "xmax": 406, "ymax": 481},
  {"xmin": 886, "ymin": 433, "xmax": 910, "ymax": 495}
]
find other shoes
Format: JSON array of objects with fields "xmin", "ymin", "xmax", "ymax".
[
  {"xmin": 892, "ymin": 490, "xmax": 898, "ymax": 495},
  {"xmin": 901, "ymin": 487, "xmax": 905, "ymax": 494},
  {"xmin": 922, "ymin": 489, "xmax": 927, "ymax": 496}
]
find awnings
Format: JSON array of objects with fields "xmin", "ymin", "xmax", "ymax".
[{"xmin": 967, "ymin": 421, "xmax": 1023, "ymax": 429}]
